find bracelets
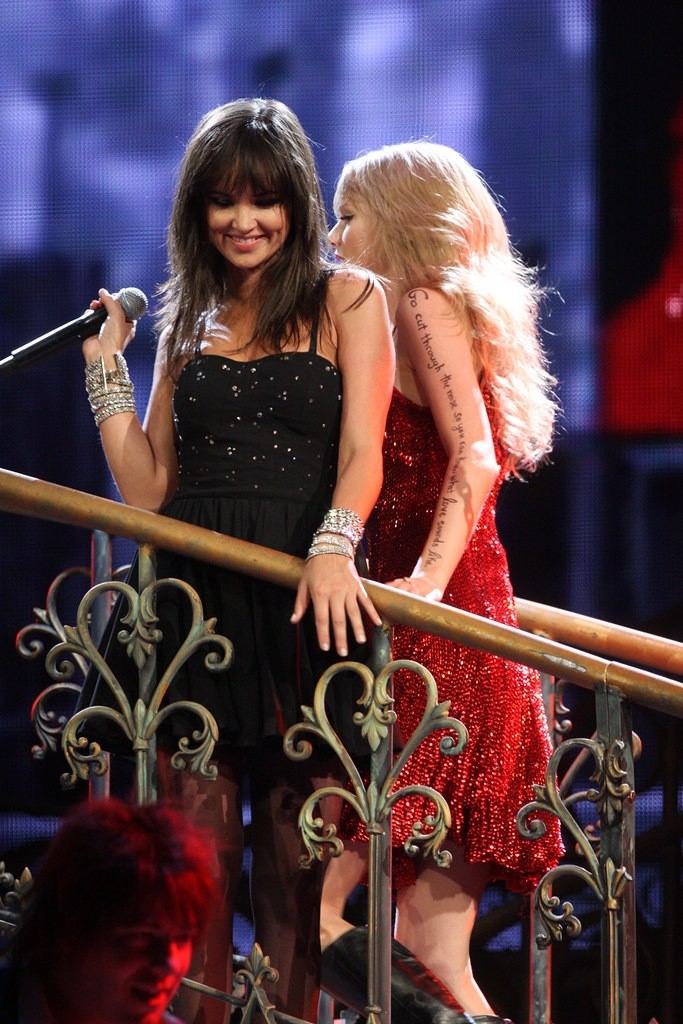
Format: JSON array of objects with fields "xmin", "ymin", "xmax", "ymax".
[
  {"xmin": 304, "ymin": 534, "xmax": 355, "ymax": 562},
  {"xmin": 85, "ymin": 353, "xmax": 136, "ymax": 427},
  {"xmin": 315, "ymin": 508, "xmax": 365, "ymax": 550}
]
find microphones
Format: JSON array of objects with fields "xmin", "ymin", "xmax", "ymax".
[{"xmin": 0, "ymin": 287, "xmax": 149, "ymax": 375}]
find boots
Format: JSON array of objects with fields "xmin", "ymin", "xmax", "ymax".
[{"xmin": 319, "ymin": 927, "xmax": 512, "ymax": 1024}]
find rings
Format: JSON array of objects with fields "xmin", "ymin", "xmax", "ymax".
[{"xmin": 404, "ymin": 575, "xmax": 412, "ymax": 584}]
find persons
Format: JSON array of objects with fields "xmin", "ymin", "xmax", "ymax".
[
  {"xmin": 81, "ymin": 99, "xmax": 395, "ymax": 1024},
  {"xmin": 320, "ymin": 141, "xmax": 568, "ymax": 1024},
  {"xmin": 0, "ymin": 797, "xmax": 228, "ymax": 1023}
]
